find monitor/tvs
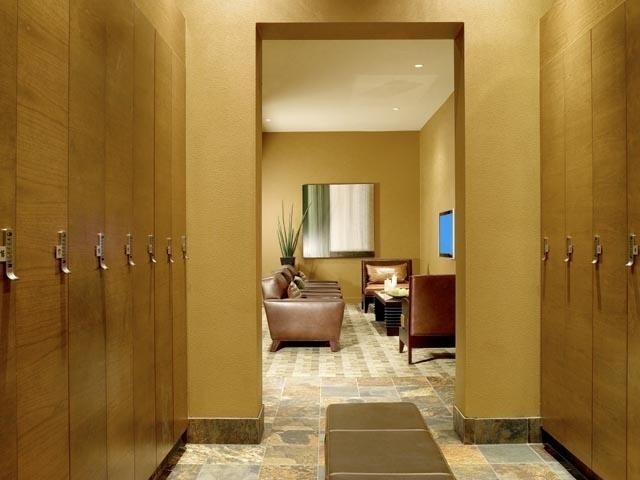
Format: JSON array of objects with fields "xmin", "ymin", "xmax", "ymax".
[{"xmin": 439, "ymin": 210, "xmax": 453, "ymax": 257}]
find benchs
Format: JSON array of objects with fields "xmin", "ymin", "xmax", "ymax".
[{"xmin": 325, "ymin": 401, "xmax": 454, "ymax": 480}]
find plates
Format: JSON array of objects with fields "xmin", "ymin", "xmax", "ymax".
[{"xmin": 386, "ymin": 289, "xmax": 404, "ymax": 299}]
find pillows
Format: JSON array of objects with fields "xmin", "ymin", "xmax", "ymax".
[
  {"xmin": 287, "ymin": 281, "xmax": 302, "ymax": 299},
  {"xmin": 367, "ymin": 264, "xmax": 408, "ymax": 283},
  {"xmin": 294, "ymin": 275, "xmax": 305, "ymax": 290},
  {"xmin": 297, "ymin": 270, "xmax": 307, "ymax": 283}
]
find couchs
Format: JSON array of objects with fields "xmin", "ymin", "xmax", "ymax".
[
  {"xmin": 282, "ymin": 264, "xmax": 339, "ymax": 286},
  {"xmin": 274, "ymin": 268, "xmax": 342, "ymax": 293},
  {"xmin": 398, "ymin": 270, "xmax": 455, "ymax": 363},
  {"xmin": 360, "ymin": 259, "xmax": 414, "ymax": 312},
  {"xmin": 263, "ymin": 273, "xmax": 344, "ymax": 353}
]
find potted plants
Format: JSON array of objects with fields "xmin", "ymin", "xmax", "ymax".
[{"xmin": 274, "ymin": 199, "xmax": 313, "ymax": 267}]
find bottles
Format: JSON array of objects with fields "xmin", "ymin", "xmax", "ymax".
[{"xmin": 384, "ymin": 273, "xmax": 398, "ymax": 292}]
